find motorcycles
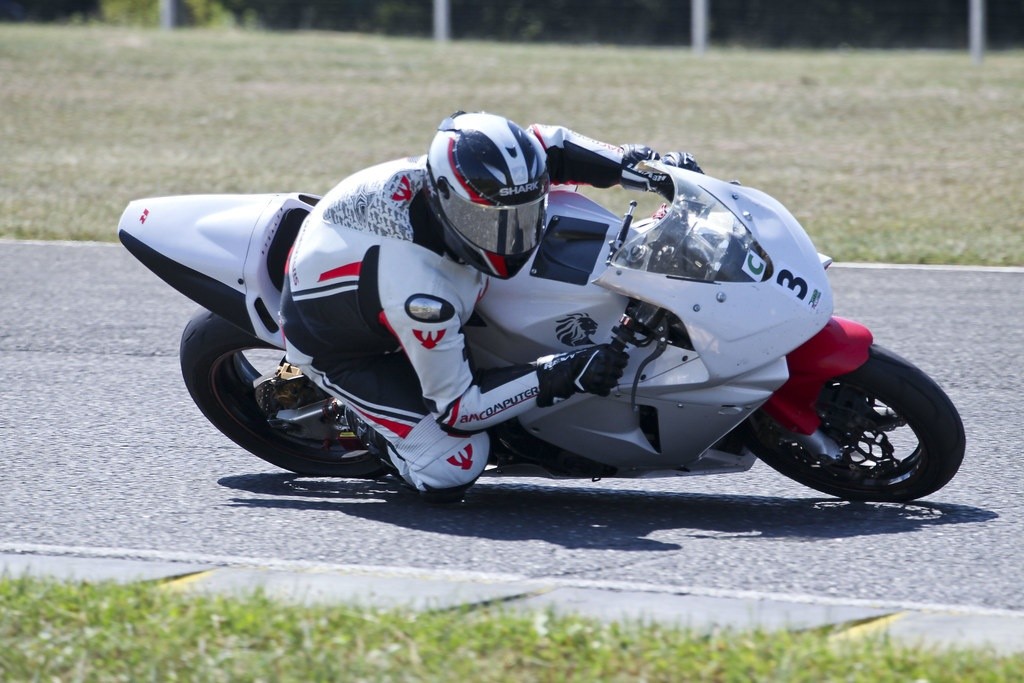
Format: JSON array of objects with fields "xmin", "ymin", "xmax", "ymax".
[{"xmin": 117, "ymin": 159, "xmax": 966, "ymax": 504}]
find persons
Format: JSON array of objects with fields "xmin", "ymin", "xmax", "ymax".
[{"xmin": 277, "ymin": 111, "xmax": 705, "ymax": 501}]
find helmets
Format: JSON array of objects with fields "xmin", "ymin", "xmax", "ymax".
[{"xmin": 422, "ymin": 110, "xmax": 551, "ymax": 281}]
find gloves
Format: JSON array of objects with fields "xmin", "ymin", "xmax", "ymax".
[
  {"xmin": 620, "ymin": 141, "xmax": 706, "ymax": 205},
  {"xmin": 536, "ymin": 343, "xmax": 630, "ymax": 407}
]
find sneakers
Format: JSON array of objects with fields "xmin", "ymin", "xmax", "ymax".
[{"xmin": 343, "ymin": 406, "xmax": 395, "ymax": 469}]
